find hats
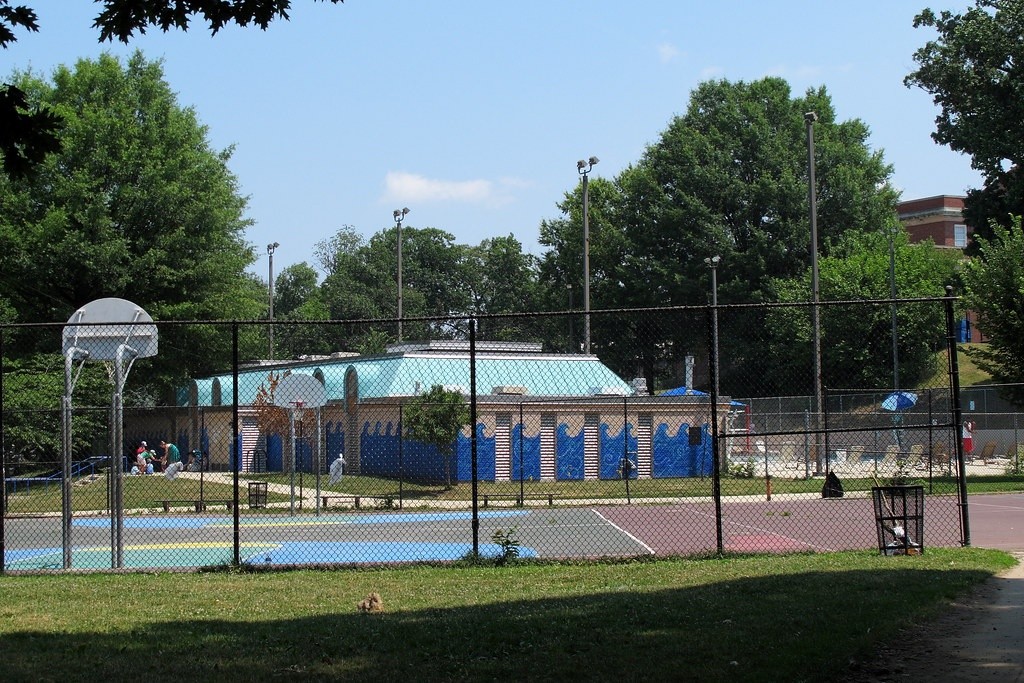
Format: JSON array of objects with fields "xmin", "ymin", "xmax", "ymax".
[
  {"xmin": 150, "ymin": 450, "xmax": 156, "ymax": 455},
  {"xmin": 141, "ymin": 440, "xmax": 147, "ymax": 446},
  {"xmin": 133, "ymin": 462, "xmax": 138, "ymax": 465},
  {"xmin": 147, "ymin": 459, "xmax": 151, "ymax": 463}
]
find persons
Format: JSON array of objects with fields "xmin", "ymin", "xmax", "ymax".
[
  {"xmin": 159, "ymin": 441, "xmax": 183, "ymax": 484},
  {"xmin": 132, "ymin": 441, "xmax": 168, "ymax": 476},
  {"xmin": 183, "ymin": 452, "xmax": 202, "ymax": 472},
  {"xmin": 963, "ymin": 416, "xmax": 978, "ymax": 465},
  {"xmin": 357, "ymin": 593, "xmax": 380, "ymax": 613}
]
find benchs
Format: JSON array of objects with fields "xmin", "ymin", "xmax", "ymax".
[
  {"xmin": 478, "ymin": 492, "xmax": 563, "ymax": 506},
  {"xmin": 153, "ymin": 499, "xmax": 240, "ymax": 512},
  {"xmin": 315, "ymin": 495, "xmax": 400, "ymax": 510}
]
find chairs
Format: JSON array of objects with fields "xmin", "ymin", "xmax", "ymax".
[
  {"xmin": 995, "ymin": 441, "xmax": 1016, "ymax": 459},
  {"xmin": 870, "ymin": 445, "xmax": 899, "ymax": 468},
  {"xmin": 846, "ymin": 446, "xmax": 865, "ymax": 469},
  {"xmin": 782, "ymin": 442, "xmax": 800, "ymax": 470},
  {"xmin": 905, "ymin": 444, "xmax": 928, "ymax": 472},
  {"xmin": 966, "ymin": 440, "xmax": 998, "ymax": 465},
  {"xmin": 732, "ymin": 446, "xmax": 750, "ymax": 456},
  {"xmin": 756, "ymin": 441, "xmax": 780, "ymax": 457},
  {"xmin": 920, "ymin": 439, "xmax": 944, "ymax": 471},
  {"xmin": 797, "ymin": 444, "xmax": 817, "ymax": 470}
]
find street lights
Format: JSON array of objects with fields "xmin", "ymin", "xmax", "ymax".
[
  {"xmin": 266, "ymin": 241, "xmax": 280, "ymax": 360},
  {"xmin": 577, "ymin": 155, "xmax": 600, "ymax": 355},
  {"xmin": 890, "ymin": 226, "xmax": 901, "ymax": 395},
  {"xmin": 394, "ymin": 205, "xmax": 412, "ymax": 343},
  {"xmin": 801, "ymin": 110, "xmax": 826, "ymax": 476},
  {"xmin": 703, "ymin": 255, "xmax": 725, "ymax": 552}
]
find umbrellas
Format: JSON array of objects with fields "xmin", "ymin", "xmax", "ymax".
[{"xmin": 882, "ymin": 390, "xmax": 917, "ymax": 412}]
